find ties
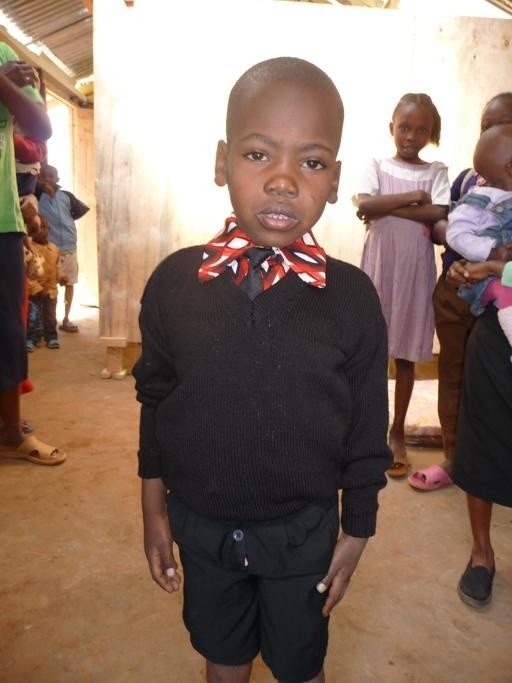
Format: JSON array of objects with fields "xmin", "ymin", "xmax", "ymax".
[{"xmin": 239, "ymin": 247, "xmax": 275, "ymax": 302}]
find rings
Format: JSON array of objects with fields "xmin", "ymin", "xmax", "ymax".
[{"xmin": 23, "ymin": 74, "xmax": 32, "ymax": 82}]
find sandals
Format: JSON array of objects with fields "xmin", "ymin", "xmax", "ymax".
[
  {"xmin": 48, "ymin": 340, "xmax": 59, "ymax": 348},
  {"xmin": 27, "ymin": 245, "xmax": 45, "ymax": 280},
  {"xmin": 26, "ymin": 338, "xmax": 36, "ymax": 352}
]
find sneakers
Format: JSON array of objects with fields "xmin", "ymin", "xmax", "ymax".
[{"xmin": 457, "ymin": 558, "xmax": 495, "ymax": 607}]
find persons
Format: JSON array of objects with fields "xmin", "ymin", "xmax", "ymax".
[
  {"xmin": 35, "ymin": 159, "xmax": 89, "ymax": 333},
  {"xmin": 351, "ymin": 87, "xmax": 448, "ymax": 478},
  {"xmin": 446, "ymin": 121, "xmax": 512, "ymax": 266},
  {"xmin": 431, "ymin": 93, "xmax": 512, "ymax": 614},
  {"xmin": 26, "ymin": 208, "xmax": 62, "ymax": 351},
  {"xmin": 134, "ymin": 53, "xmax": 388, "ymax": 680},
  {"xmin": 1, "ymin": 35, "xmax": 69, "ymax": 464}
]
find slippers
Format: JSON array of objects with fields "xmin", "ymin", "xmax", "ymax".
[
  {"xmin": 387, "ymin": 452, "xmax": 408, "ymax": 477},
  {"xmin": 0, "ymin": 435, "xmax": 67, "ymax": 465},
  {"xmin": 59, "ymin": 323, "xmax": 78, "ymax": 332},
  {"xmin": 407, "ymin": 465, "xmax": 453, "ymax": 491}
]
